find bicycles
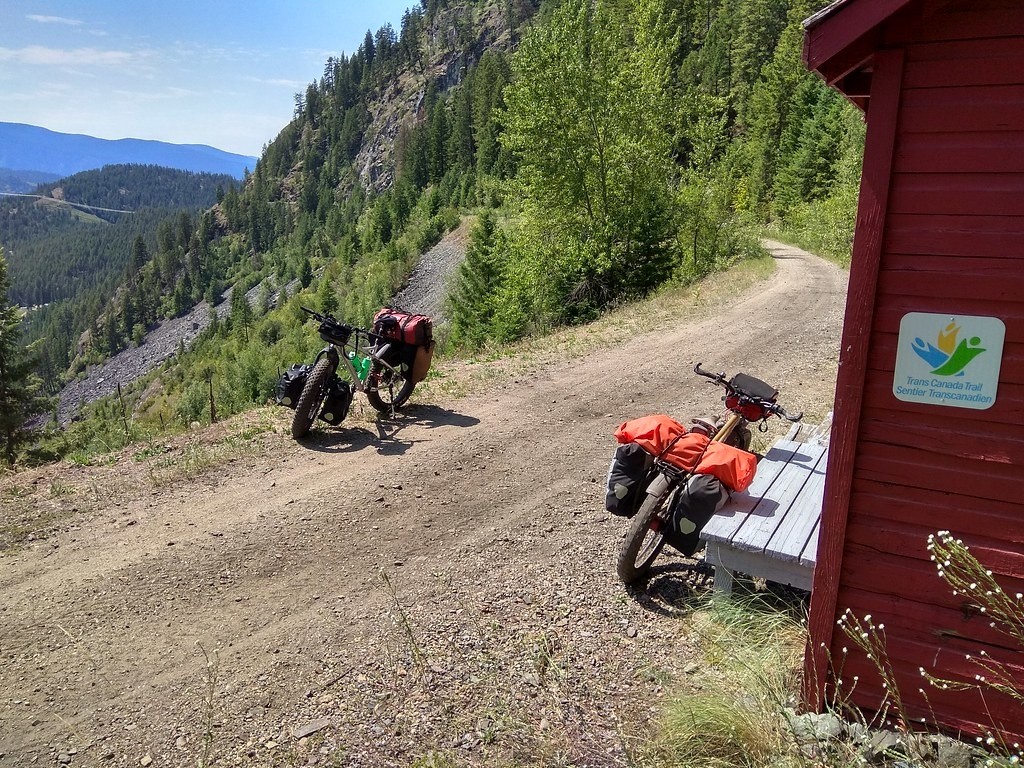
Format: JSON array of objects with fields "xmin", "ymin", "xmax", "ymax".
[
  {"xmin": 617, "ymin": 363, "xmax": 804, "ymax": 583},
  {"xmin": 291, "ymin": 305, "xmax": 416, "ymax": 440}
]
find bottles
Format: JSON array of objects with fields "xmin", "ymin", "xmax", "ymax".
[
  {"xmin": 348, "ymin": 351, "xmax": 363, "ymax": 372},
  {"xmin": 357, "ymin": 355, "xmax": 371, "ymax": 381}
]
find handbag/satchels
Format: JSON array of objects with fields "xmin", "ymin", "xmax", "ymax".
[
  {"xmin": 276, "ymin": 362, "xmax": 312, "ymax": 410},
  {"xmin": 371, "ymin": 307, "xmax": 435, "ymax": 383},
  {"xmin": 318, "ymin": 377, "xmax": 356, "ymax": 425},
  {"xmin": 604, "ymin": 416, "xmax": 756, "ymax": 558}
]
